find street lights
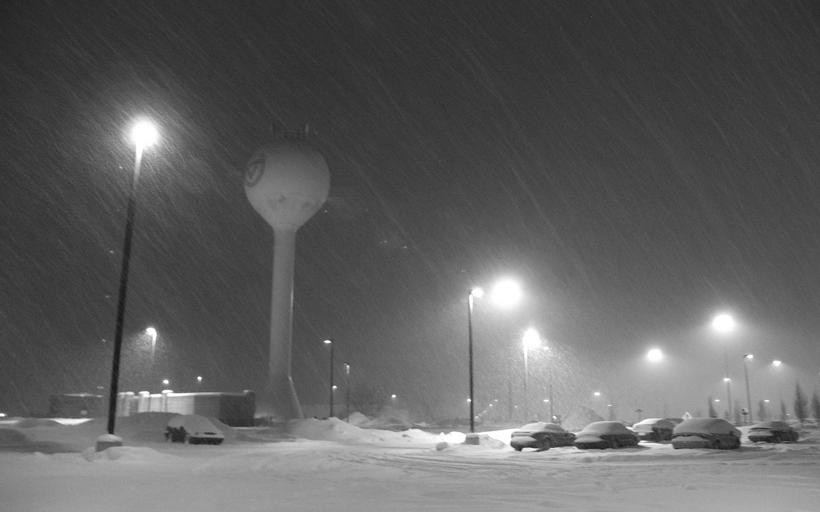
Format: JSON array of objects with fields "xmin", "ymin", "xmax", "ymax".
[
  {"xmin": 594, "ymin": 390, "xmax": 614, "ymax": 421},
  {"xmin": 146, "ymin": 326, "xmax": 157, "ymax": 394},
  {"xmin": 321, "ymin": 336, "xmax": 351, "ymax": 422},
  {"xmin": 723, "ymin": 353, "xmax": 753, "ymax": 425},
  {"xmin": 97, "ymin": 116, "xmax": 155, "ymax": 451},
  {"xmin": 522, "ymin": 327, "xmax": 538, "ymax": 423},
  {"xmin": 467, "ymin": 281, "xmax": 517, "ymax": 434},
  {"xmin": 714, "ymin": 313, "xmax": 734, "ymax": 421}
]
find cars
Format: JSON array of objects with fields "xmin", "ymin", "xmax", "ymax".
[
  {"xmin": 511, "ymin": 417, "xmax": 800, "ymax": 451},
  {"xmin": 171, "ymin": 414, "xmax": 224, "ymax": 444}
]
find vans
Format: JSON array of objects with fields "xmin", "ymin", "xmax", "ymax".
[{"xmin": 62, "ymin": 394, "xmax": 104, "ymax": 418}]
